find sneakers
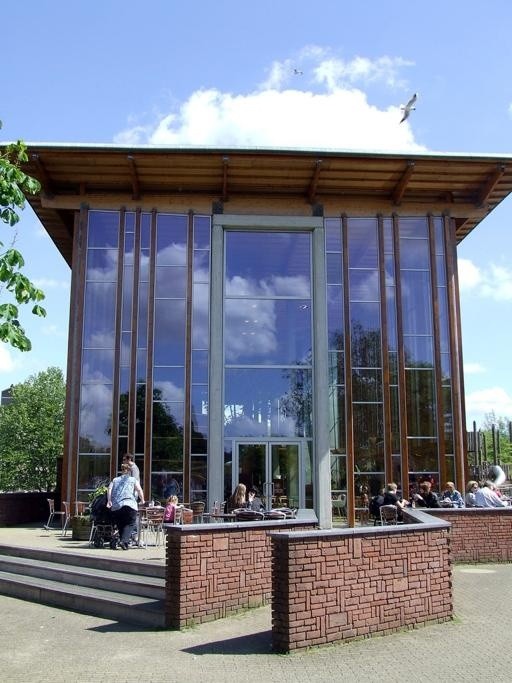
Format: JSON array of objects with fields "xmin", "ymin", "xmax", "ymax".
[{"xmin": 119, "ymin": 542, "xmax": 128, "ymax": 550}]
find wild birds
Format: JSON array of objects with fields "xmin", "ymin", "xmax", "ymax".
[
  {"xmin": 292, "ymin": 68, "xmax": 303, "ymax": 76},
  {"xmin": 399, "ymin": 93, "xmax": 417, "ymax": 125}
]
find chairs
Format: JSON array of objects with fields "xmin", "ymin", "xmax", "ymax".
[
  {"xmin": 44, "ymin": 495, "xmax": 297, "ymax": 549},
  {"xmin": 331, "ymin": 493, "xmax": 403, "ymax": 525}
]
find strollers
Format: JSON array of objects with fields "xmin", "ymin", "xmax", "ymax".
[{"xmin": 88, "ymin": 494, "xmax": 145, "ymax": 550}]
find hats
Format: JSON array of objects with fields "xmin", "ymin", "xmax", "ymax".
[
  {"xmin": 466, "ymin": 481, "xmax": 478, "ymax": 492},
  {"xmin": 249, "ymin": 485, "xmax": 257, "ymax": 492}
]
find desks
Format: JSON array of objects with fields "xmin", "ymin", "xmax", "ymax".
[{"xmin": 439, "ymin": 500, "xmax": 464, "ymax": 508}]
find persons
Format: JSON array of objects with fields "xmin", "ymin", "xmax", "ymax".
[
  {"xmin": 247, "ymin": 486, "xmax": 265, "ymax": 513},
  {"xmin": 440, "ymin": 479, "xmax": 464, "ymax": 508},
  {"xmin": 123, "ymin": 452, "xmax": 140, "ymax": 499},
  {"xmin": 120, "ymin": 463, "xmax": 131, "ymax": 477},
  {"xmin": 384, "ymin": 482, "xmax": 409, "ymax": 520},
  {"xmin": 475, "ymin": 478, "xmax": 509, "ymax": 507},
  {"xmin": 223, "ymin": 482, "xmax": 247, "ymax": 523},
  {"xmin": 104, "ymin": 462, "xmax": 145, "ymax": 550},
  {"xmin": 163, "ymin": 475, "xmax": 179, "ymax": 499},
  {"xmin": 373, "ymin": 486, "xmax": 385, "ymax": 523},
  {"xmin": 413, "ymin": 480, "xmax": 442, "ymax": 508},
  {"xmin": 465, "ymin": 479, "xmax": 478, "ymax": 508},
  {"xmin": 91, "ymin": 491, "xmax": 115, "ymax": 534},
  {"xmin": 162, "ymin": 495, "xmax": 179, "ymax": 525}
]
